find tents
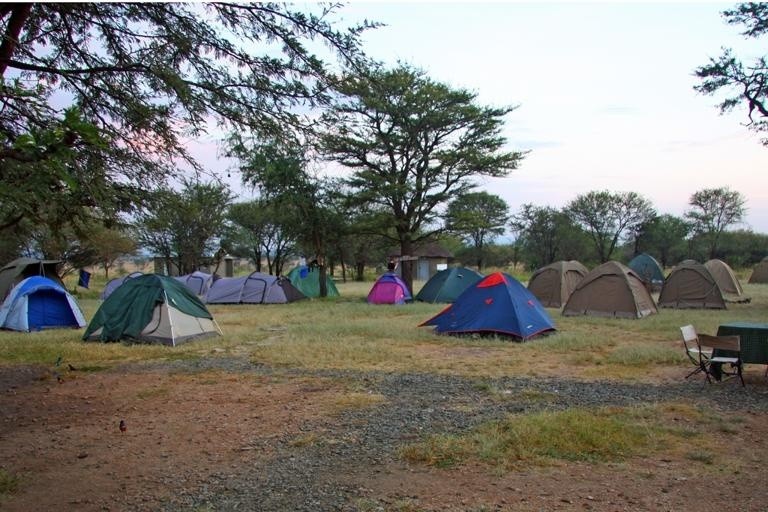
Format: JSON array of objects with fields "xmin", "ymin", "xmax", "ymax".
[
  {"xmin": 419, "ymin": 271, "xmax": 555, "ymax": 338},
  {"xmin": 81, "ymin": 273, "xmax": 222, "ymax": 348},
  {"xmin": 748, "ymin": 256, "xmax": 768, "ymax": 285},
  {"xmin": 627, "ymin": 254, "xmax": 665, "ymax": 282},
  {"xmin": 0, "ymin": 256, "xmax": 67, "ymax": 303},
  {"xmin": 702, "ymin": 259, "xmax": 744, "ymax": 297},
  {"xmin": 416, "ymin": 266, "xmax": 485, "ymax": 304},
  {"xmin": 528, "ymin": 260, "xmax": 589, "ymax": 307},
  {"xmin": 660, "ymin": 256, "xmax": 727, "ymax": 310},
  {"xmin": 561, "ymin": 260, "xmax": 658, "ymax": 319},
  {"xmin": 366, "ymin": 270, "xmax": 412, "ymax": 304},
  {"xmin": 84, "ymin": 262, "xmax": 342, "ymax": 304},
  {"xmin": 0, "ymin": 276, "xmax": 88, "ymax": 333}
]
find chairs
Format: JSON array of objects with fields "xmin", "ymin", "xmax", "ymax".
[
  {"xmin": 697, "ymin": 333, "xmax": 745, "ymax": 392},
  {"xmin": 680, "ymin": 324, "xmax": 729, "ymax": 379}
]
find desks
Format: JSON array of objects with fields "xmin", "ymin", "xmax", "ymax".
[{"xmin": 709, "ymin": 321, "xmax": 768, "ymax": 382}]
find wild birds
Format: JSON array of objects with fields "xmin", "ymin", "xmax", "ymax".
[
  {"xmin": 57, "ymin": 377, "xmax": 65, "ymax": 384},
  {"xmin": 68, "ymin": 363, "xmax": 76, "ymax": 371},
  {"xmin": 119, "ymin": 420, "xmax": 127, "ymax": 436}
]
